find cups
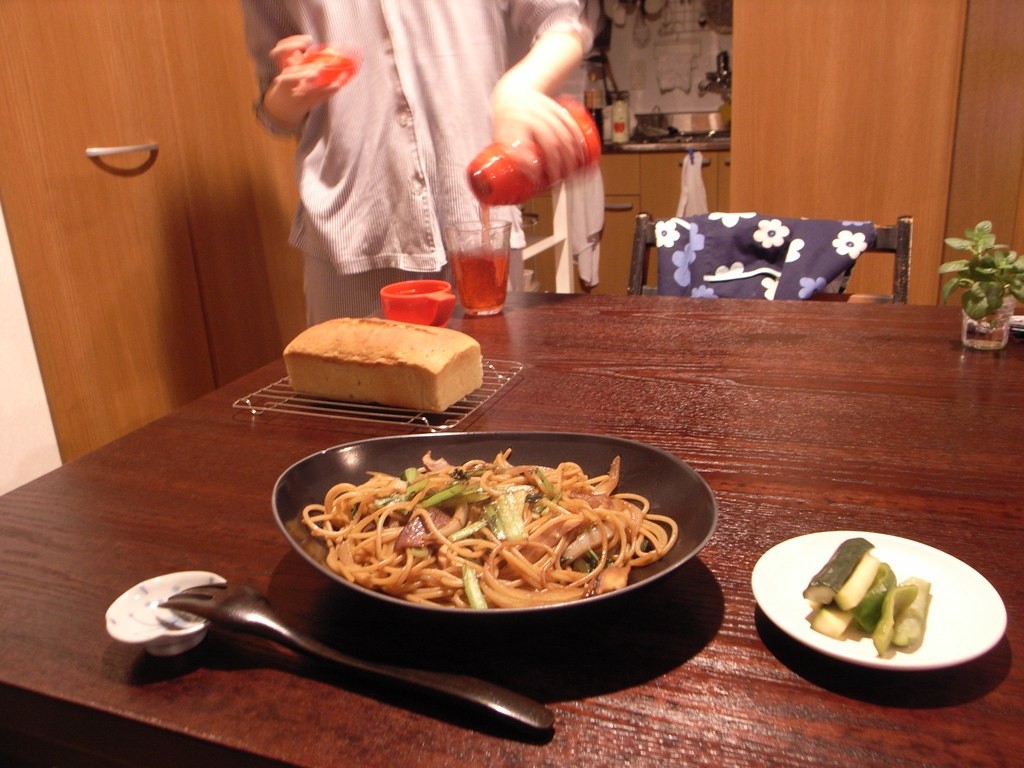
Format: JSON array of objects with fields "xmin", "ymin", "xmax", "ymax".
[
  {"xmin": 444, "ymin": 220, "xmax": 512, "ymax": 316},
  {"xmin": 380, "ymin": 280, "xmax": 456, "ymax": 328}
]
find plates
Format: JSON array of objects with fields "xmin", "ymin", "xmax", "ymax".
[{"xmin": 751, "ymin": 531, "xmax": 1007, "ymax": 671}]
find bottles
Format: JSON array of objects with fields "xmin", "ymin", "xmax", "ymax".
[
  {"xmin": 468, "ymin": 94, "xmax": 602, "ymax": 205},
  {"xmin": 612, "ymin": 93, "xmax": 628, "ymax": 143}
]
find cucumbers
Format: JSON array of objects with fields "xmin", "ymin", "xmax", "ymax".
[
  {"xmin": 803, "ymin": 537, "xmax": 879, "ymax": 638},
  {"xmin": 891, "ymin": 577, "xmax": 931, "ymax": 645}
]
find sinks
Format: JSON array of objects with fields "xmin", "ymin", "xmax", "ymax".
[{"xmin": 657, "ymin": 136, "xmax": 713, "ymax": 145}]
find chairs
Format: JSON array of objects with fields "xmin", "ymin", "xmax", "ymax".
[{"xmin": 627, "ymin": 211, "xmax": 913, "ymax": 303}]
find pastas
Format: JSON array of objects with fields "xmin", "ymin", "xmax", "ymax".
[{"xmin": 300, "ymin": 447, "xmax": 679, "ymax": 608}]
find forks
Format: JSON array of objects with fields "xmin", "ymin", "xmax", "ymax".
[{"xmin": 157, "ymin": 584, "xmax": 554, "ymax": 729}]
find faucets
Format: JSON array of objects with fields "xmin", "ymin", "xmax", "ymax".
[{"xmin": 680, "ymin": 130, "xmax": 700, "ymax": 136}]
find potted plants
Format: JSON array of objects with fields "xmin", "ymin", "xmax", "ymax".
[{"xmin": 937, "ymin": 221, "xmax": 1024, "ymax": 351}]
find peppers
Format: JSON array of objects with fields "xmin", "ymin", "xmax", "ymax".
[{"xmin": 852, "ymin": 562, "xmax": 919, "ymax": 654}]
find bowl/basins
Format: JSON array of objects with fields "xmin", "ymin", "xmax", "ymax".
[
  {"xmin": 272, "ymin": 431, "xmax": 718, "ymax": 613},
  {"xmin": 105, "ymin": 570, "xmax": 227, "ymax": 656}
]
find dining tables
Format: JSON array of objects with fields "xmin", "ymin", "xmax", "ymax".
[{"xmin": 0, "ymin": 292, "xmax": 1024, "ymax": 768}]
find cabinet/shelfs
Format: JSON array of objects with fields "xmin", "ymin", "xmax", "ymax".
[
  {"xmin": 0, "ymin": 0, "xmax": 306, "ymax": 467},
  {"xmin": 522, "ymin": 154, "xmax": 641, "ymax": 293},
  {"xmin": 640, "ymin": 151, "xmax": 731, "ymax": 287},
  {"xmin": 730, "ymin": 1, "xmax": 1024, "ymax": 308}
]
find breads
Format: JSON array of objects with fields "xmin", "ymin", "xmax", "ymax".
[{"xmin": 283, "ymin": 318, "xmax": 483, "ymax": 411}]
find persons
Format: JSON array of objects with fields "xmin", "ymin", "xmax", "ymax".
[{"xmin": 242, "ymin": 0, "xmax": 605, "ymax": 327}]
